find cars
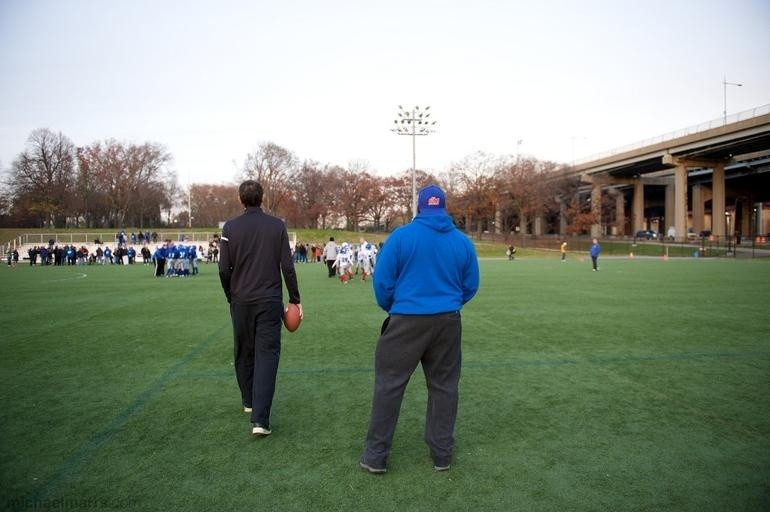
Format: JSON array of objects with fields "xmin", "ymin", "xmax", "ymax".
[
  {"xmin": 637, "ymin": 231, "xmax": 663, "ymax": 241},
  {"xmin": 755, "ymin": 233, "xmax": 770, "ymax": 245}
]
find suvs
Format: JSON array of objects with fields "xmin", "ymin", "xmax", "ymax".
[
  {"xmin": 668, "ymin": 226, "xmax": 697, "ymax": 242},
  {"xmin": 688, "ymin": 227, "xmax": 711, "ymax": 240}
]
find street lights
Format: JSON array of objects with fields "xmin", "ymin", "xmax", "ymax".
[
  {"xmin": 724, "ymin": 82, "xmax": 742, "ymax": 126},
  {"xmin": 391, "ymin": 104, "xmax": 437, "ymax": 220},
  {"xmin": 724, "ymin": 211, "xmax": 732, "ymax": 252}
]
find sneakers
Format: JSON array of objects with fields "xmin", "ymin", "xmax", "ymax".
[
  {"xmin": 359, "ymin": 456, "xmax": 386, "ymax": 474},
  {"xmin": 435, "ymin": 463, "xmax": 450, "ymax": 472},
  {"xmin": 242, "ymin": 406, "xmax": 252, "ymax": 412},
  {"xmin": 251, "ymin": 422, "xmax": 272, "ymax": 435}
]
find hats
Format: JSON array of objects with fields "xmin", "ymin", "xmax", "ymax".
[{"xmin": 419, "ymin": 185, "xmax": 445, "ymax": 215}]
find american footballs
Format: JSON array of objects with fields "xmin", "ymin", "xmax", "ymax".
[{"xmin": 282, "ymin": 304, "xmax": 301, "ymax": 332}]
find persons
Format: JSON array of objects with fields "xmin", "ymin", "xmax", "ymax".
[
  {"xmin": 291, "ymin": 237, "xmax": 384, "ymax": 288},
  {"xmin": 560, "ymin": 241, "xmax": 568, "ymax": 263},
  {"xmin": 508, "ymin": 244, "xmax": 516, "ymax": 260},
  {"xmin": 590, "ymin": 238, "xmax": 601, "ymax": 272},
  {"xmin": 218, "ymin": 180, "xmax": 304, "ymax": 435},
  {"xmin": 6, "ymin": 230, "xmax": 221, "ymax": 278},
  {"xmin": 360, "ymin": 185, "xmax": 480, "ymax": 474}
]
